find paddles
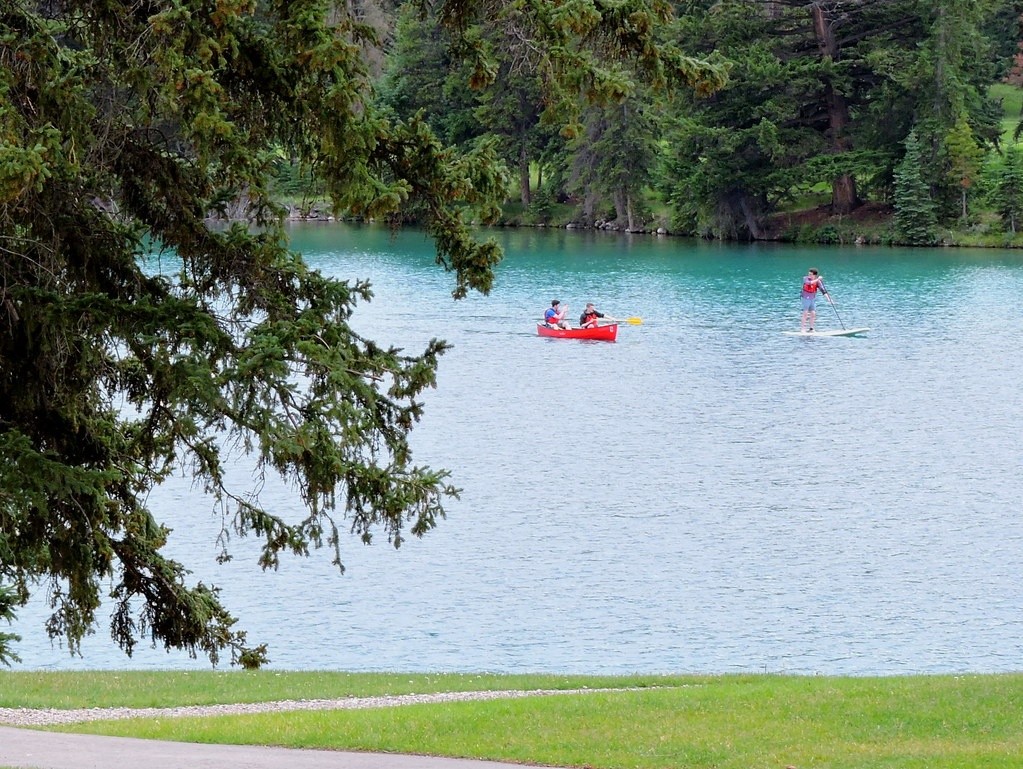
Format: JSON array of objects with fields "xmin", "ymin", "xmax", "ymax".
[
  {"xmin": 531, "ymin": 317, "xmax": 575, "ymax": 322},
  {"xmin": 820, "ymin": 279, "xmax": 846, "ymax": 330},
  {"xmin": 590, "ymin": 317, "xmax": 642, "ymax": 326}
]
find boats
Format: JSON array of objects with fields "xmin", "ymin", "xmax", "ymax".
[{"xmin": 536, "ymin": 322, "xmax": 619, "ymax": 341}]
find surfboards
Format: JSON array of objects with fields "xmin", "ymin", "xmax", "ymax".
[{"xmin": 780, "ymin": 327, "xmax": 871, "ymax": 336}]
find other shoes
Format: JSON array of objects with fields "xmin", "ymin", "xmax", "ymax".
[
  {"xmin": 801, "ymin": 328, "xmax": 806, "ymax": 334},
  {"xmin": 810, "ymin": 329, "xmax": 815, "ymax": 333}
]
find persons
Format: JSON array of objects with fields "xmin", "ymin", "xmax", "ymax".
[
  {"xmin": 799, "ymin": 268, "xmax": 833, "ymax": 334},
  {"xmin": 543, "ymin": 299, "xmax": 572, "ymax": 330},
  {"xmin": 579, "ymin": 302, "xmax": 618, "ymax": 330}
]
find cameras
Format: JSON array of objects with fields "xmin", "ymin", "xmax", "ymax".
[{"xmin": 565, "ymin": 303, "xmax": 568, "ymax": 307}]
